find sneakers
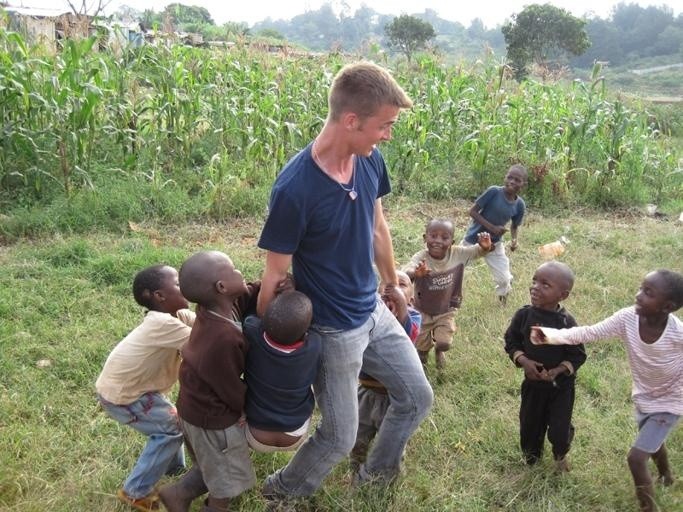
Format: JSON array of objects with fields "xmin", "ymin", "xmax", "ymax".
[
  {"xmin": 118, "ymin": 487, "xmax": 160, "ymax": 512},
  {"xmin": 259, "ymin": 465, "xmax": 317, "ymax": 512},
  {"xmin": 158, "ymin": 483, "xmax": 193, "ymax": 512},
  {"xmin": 350, "ymin": 462, "xmax": 395, "ymax": 493}
]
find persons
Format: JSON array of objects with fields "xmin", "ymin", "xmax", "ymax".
[
  {"xmin": 504, "ymin": 261, "xmax": 588, "ymax": 465},
  {"xmin": 245, "ymin": 273, "xmax": 324, "ymax": 456},
  {"xmin": 530, "ymin": 268, "xmax": 682, "ymax": 511},
  {"xmin": 158, "ymin": 251, "xmax": 257, "ymax": 512},
  {"xmin": 257, "ymin": 61, "xmax": 434, "ymax": 502},
  {"xmin": 400, "ymin": 217, "xmax": 496, "ymax": 370},
  {"xmin": 459, "ymin": 165, "xmax": 526, "ymax": 306},
  {"xmin": 359, "ymin": 271, "xmax": 436, "ymax": 466},
  {"xmin": 95, "ymin": 265, "xmax": 197, "ymax": 511}
]
